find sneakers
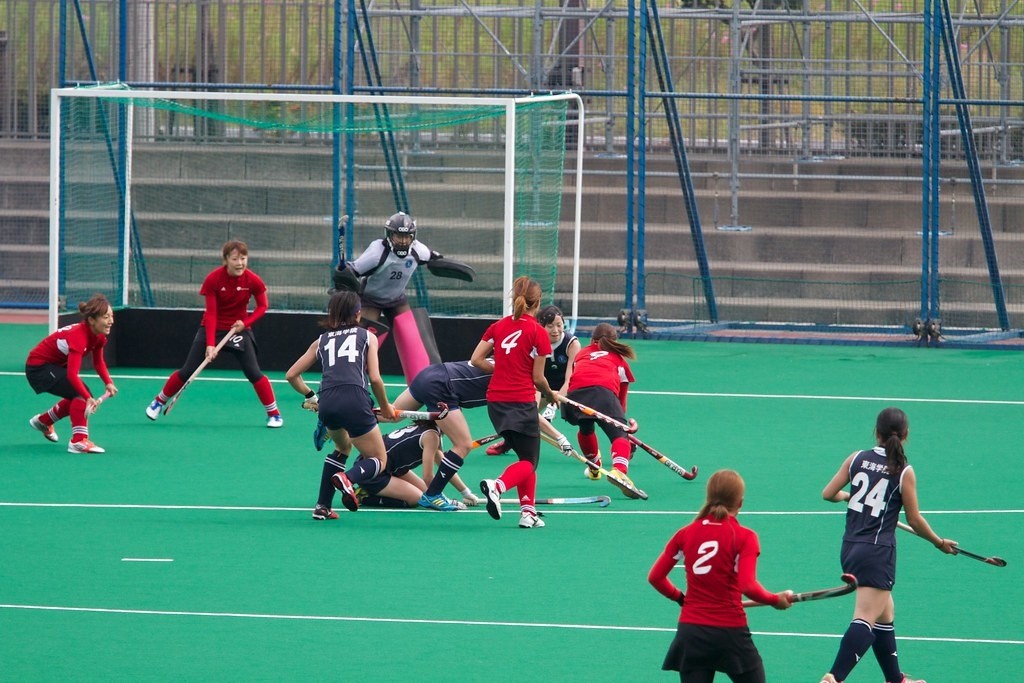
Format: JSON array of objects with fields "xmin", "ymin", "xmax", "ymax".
[
  {"xmin": 68, "ymin": 438, "xmax": 105, "ymax": 454},
  {"xmin": 418, "ymin": 492, "xmax": 459, "ymax": 511},
  {"xmin": 519, "ymin": 512, "xmax": 545, "ymax": 528},
  {"xmin": 584, "ymin": 466, "xmax": 601, "ymax": 480},
  {"xmin": 331, "ymin": 471, "xmax": 358, "ymax": 512},
  {"xmin": 33, "ymin": 415, "xmax": 58, "ymax": 442},
  {"xmin": 606, "ymin": 466, "xmax": 640, "ymax": 499},
  {"xmin": 354, "ymin": 488, "xmax": 370, "ymax": 507},
  {"xmin": 146, "ymin": 399, "xmax": 163, "ymax": 420},
  {"xmin": 478, "ymin": 478, "xmax": 502, "ymax": 520},
  {"xmin": 313, "ymin": 421, "xmax": 334, "ymax": 452},
  {"xmin": 265, "ymin": 414, "xmax": 284, "ymax": 427},
  {"xmin": 820, "ymin": 673, "xmax": 844, "ymax": 683},
  {"xmin": 487, "ymin": 439, "xmax": 511, "ymax": 455},
  {"xmin": 885, "ymin": 672, "xmax": 926, "ymax": 683},
  {"xmin": 312, "ymin": 504, "xmax": 339, "ymax": 520},
  {"xmin": 629, "ymin": 440, "xmax": 637, "ymax": 460}
]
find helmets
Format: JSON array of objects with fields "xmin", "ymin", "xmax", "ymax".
[{"xmin": 384, "ymin": 212, "xmax": 417, "ymax": 251}]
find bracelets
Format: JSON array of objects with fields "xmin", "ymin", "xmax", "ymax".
[{"xmin": 936, "ymin": 538, "xmax": 945, "ymax": 548}]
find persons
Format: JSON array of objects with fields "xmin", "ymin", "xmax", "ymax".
[
  {"xmin": 314, "ymin": 358, "xmax": 581, "ymax": 512},
  {"xmin": 353, "ymin": 421, "xmax": 478, "ymax": 508},
  {"xmin": 471, "ymin": 277, "xmax": 560, "ymax": 528},
  {"xmin": 334, "ymin": 212, "xmax": 477, "ymax": 385},
  {"xmin": 648, "ymin": 471, "xmax": 795, "ymax": 683},
  {"xmin": 823, "ymin": 407, "xmax": 958, "ymax": 683},
  {"xmin": 567, "ymin": 323, "xmax": 639, "ymax": 498},
  {"xmin": 26, "ymin": 295, "xmax": 117, "ymax": 453},
  {"xmin": 285, "ymin": 291, "xmax": 395, "ymax": 520},
  {"xmin": 146, "ymin": 242, "xmax": 283, "ymax": 427},
  {"xmin": 485, "ymin": 305, "xmax": 581, "ymax": 456}
]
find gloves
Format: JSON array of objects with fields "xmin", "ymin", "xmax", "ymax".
[
  {"xmin": 449, "ymin": 498, "xmax": 467, "ymax": 509},
  {"xmin": 460, "ymin": 487, "xmax": 479, "ymax": 506},
  {"xmin": 541, "ymin": 403, "xmax": 556, "ymax": 422},
  {"xmin": 676, "ymin": 593, "xmax": 686, "ymax": 607},
  {"xmin": 557, "ymin": 433, "xmax": 580, "ymax": 460},
  {"xmin": 305, "ymin": 391, "xmax": 319, "ymax": 412}
]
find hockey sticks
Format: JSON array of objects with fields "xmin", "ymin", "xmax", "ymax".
[
  {"xmin": 301, "ymin": 400, "xmax": 447, "ymax": 421},
  {"xmin": 896, "ymin": 521, "xmax": 1007, "ymax": 568},
  {"xmin": 462, "ymin": 495, "xmax": 612, "ymax": 508},
  {"xmin": 541, "ymin": 393, "xmax": 639, "ymax": 434},
  {"xmin": 540, "ymin": 433, "xmax": 649, "ymax": 500},
  {"xmin": 163, "ymin": 326, "xmax": 237, "ymax": 416},
  {"xmin": 742, "ymin": 573, "xmax": 859, "ymax": 607},
  {"xmin": 472, "ymin": 433, "xmax": 502, "ymax": 448},
  {"xmin": 627, "ymin": 433, "xmax": 699, "ymax": 481},
  {"xmin": 337, "ymin": 214, "xmax": 349, "ymax": 271},
  {"xmin": 83, "ymin": 390, "xmax": 111, "ymax": 419}
]
